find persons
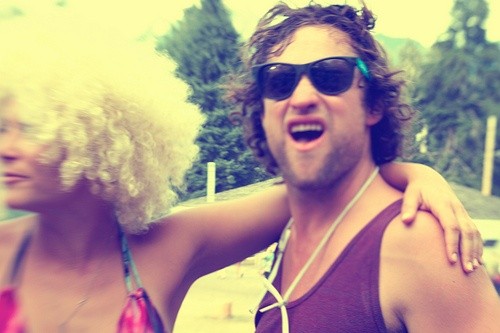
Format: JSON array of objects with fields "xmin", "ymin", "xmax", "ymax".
[
  {"xmin": 216, "ymin": 0, "xmax": 500, "ymax": 333},
  {"xmin": 0, "ymin": 36, "xmax": 484, "ymax": 333}
]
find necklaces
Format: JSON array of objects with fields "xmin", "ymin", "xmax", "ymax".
[{"xmin": 52, "ymin": 228, "xmax": 115, "ymax": 333}]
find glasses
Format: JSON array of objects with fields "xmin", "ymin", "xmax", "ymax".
[{"xmin": 251, "ymin": 56, "xmax": 369, "ymax": 102}]
